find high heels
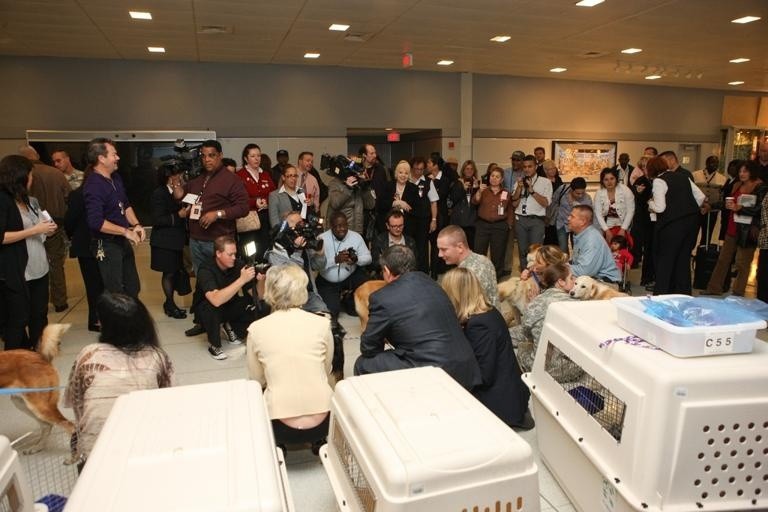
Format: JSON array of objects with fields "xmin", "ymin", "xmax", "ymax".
[{"xmin": 163, "ymin": 302, "xmax": 188, "ymax": 319}]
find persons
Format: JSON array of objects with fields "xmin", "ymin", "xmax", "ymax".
[
  {"xmin": 1, "ymin": 136, "xmax": 146, "ymax": 352},
  {"xmin": 458, "ymin": 146, "xmax": 562, "ymax": 281},
  {"xmin": 594, "ymin": 146, "xmax": 768, "ymax": 303},
  {"xmin": 436, "ymin": 226, "xmax": 498, "ymax": 306},
  {"xmin": 436, "ymin": 266, "xmax": 532, "ymax": 429},
  {"xmin": 510, "ymin": 177, "xmax": 621, "ymax": 382},
  {"xmin": 60, "ymin": 285, "xmax": 174, "ymax": 480},
  {"xmin": 243, "ymin": 259, "xmax": 336, "ymax": 455},
  {"xmin": 148, "ymin": 141, "xmax": 328, "ymax": 360},
  {"xmin": 313, "ymin": 144, "xmax": 470, "ymax": 315},
  {"xmin": 353, "ymin": 243, "xmax": 485, "ymax": 404}
]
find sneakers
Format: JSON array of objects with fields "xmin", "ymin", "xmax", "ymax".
[
  {"xmin": 222, "ymin": 322, "xmax": 243, "ymax": 345},
  {"xmin": 208, "ymin": 343, "xmax": 227, "ymax": 360},
  {"xmin": 54, "ymin": 304, "xmax": 69, "ymax": 312},
  {"xmin": 88, "ymin": 323, "xmax": 104, "ymax": 333}
]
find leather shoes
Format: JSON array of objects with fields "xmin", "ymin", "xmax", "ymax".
[
  {"xmin": 340, "ymin": 301, "xmax": 360, "ymax": 317},
  {"xmin": 184, "ymin": 323, "xmax": 207, "ymax": 336}
]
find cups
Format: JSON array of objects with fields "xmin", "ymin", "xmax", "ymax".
[{"xmin": 725, "ymin": 197, "xmax": 736, "ymax": 210}]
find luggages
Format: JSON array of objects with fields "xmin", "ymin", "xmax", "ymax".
[{"xmin": 693, "ymin": 209, "xmax": 732, "ymax": 293}]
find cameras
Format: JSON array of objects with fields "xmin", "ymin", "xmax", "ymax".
[
  {"xmin": 245, "ymin": 261, "xmax": 271, "ymax": 279},
  {"xmin": 523, "ymin": 176, "xmax": 531, "ymax": 188},
  {"xmin": 347, "ymin": 247, "xmax": 358, "ymax": 262}
]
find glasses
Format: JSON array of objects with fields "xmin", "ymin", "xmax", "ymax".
[{"xmin": 389, "ymin": 224, "xmax": 405, "ymax": 229}]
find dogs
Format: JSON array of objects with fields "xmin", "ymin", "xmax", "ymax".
[
  {"xmin": 498, "ymin": 242, "xmax": 547, "ymax": 327},
  {"xmin": 0, "ymin": 322, "xmax": 80, "ymax": 465},
  {"xmin": 353, "ymin": 280, "xmax": 387, "ymax": 334},
  {"xmin": 568, "ymin": 275, "xmax": 630, "ymax": 301}
]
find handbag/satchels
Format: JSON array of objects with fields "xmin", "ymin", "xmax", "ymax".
[{"xmin": 549, "ymin": 202, "xmax": 560, "ymax": 226}]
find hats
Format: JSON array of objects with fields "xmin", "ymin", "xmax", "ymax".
[
  {"xmin": 510, "ymin": 150, "xmax": 526, "ymax": 160},
  {"xmin": 276, "ymin": 149, "xmax": 289, "ymax": 158}
]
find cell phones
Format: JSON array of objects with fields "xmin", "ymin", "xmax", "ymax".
[
  {"xmin": 481, "ymin": 176, "xmax": 488, "ymax": 186},
  {"xmin": 466, "ymin": 176, "xmax": 473, "ymax": 185}
]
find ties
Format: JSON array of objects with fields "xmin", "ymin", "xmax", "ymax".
[{"xmin": 301, "ymin": 173, "xmax": 308, "ymax": 195}]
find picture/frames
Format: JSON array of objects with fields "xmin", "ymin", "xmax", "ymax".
[{"xmin": 550, "ymin": 140, "xmax": 619, "ymax": 184}]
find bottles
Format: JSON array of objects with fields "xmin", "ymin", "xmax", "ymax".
[{"xmin": 32, "ymin": 494, "xmax": 67, "ymax": 512}]
